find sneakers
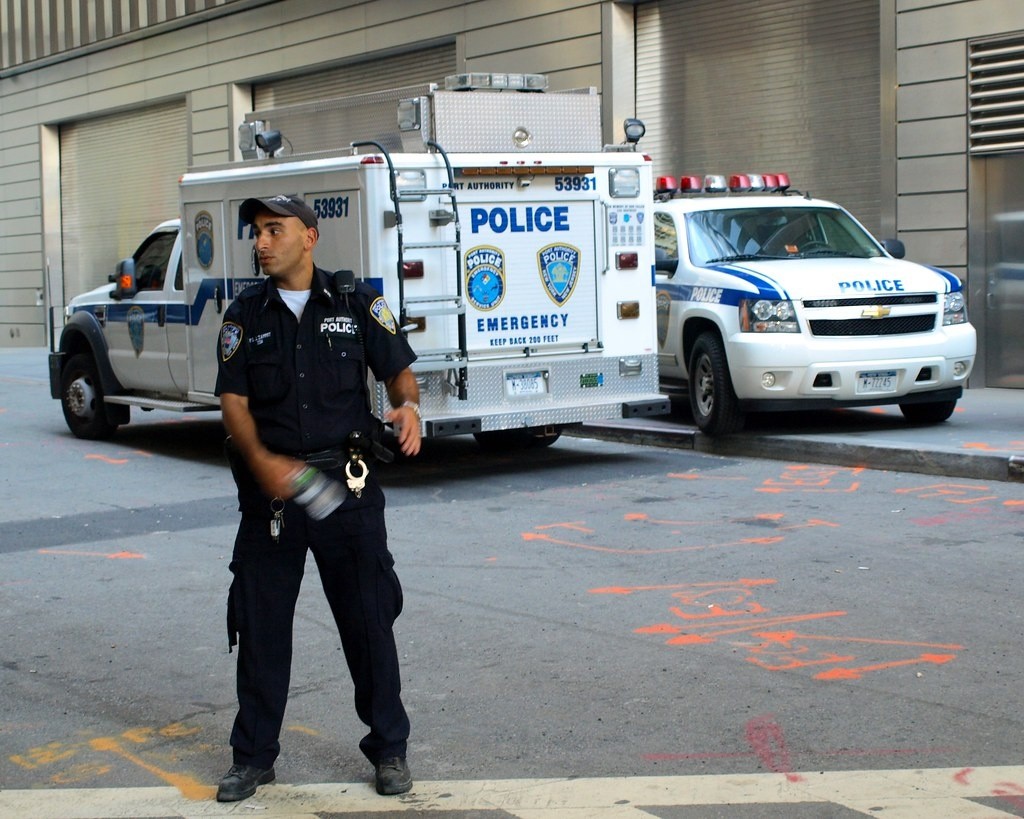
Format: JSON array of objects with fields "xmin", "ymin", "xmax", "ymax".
[
  {"xmin": 373, "ymin": 753, "xmax": 414, "ymax": 795},
  {"xmin": 217, "ymin": 759, "xmax": 276, "ymax": 801}
]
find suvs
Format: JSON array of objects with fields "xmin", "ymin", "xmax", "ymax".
[{"xmin": 653, "ymin": 174, "xmax": 977, "ymax": 430}]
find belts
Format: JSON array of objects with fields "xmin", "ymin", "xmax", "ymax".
[{"xmin": 291, "ymin": 447, "xmax": 346, "ymax": 475}]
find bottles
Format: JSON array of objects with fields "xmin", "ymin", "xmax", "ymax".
[{"xmin": 282, "ymin": 462, "xmax": 344, "ymax": 519}]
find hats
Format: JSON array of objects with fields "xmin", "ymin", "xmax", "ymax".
[{"xmin": 238, "ymin": 195, "xmax": 321, "ymax": 240}]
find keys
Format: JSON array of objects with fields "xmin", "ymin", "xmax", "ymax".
[{"xmin": 272, "ymin": 510, "xmax": 286, "ymax": 528}]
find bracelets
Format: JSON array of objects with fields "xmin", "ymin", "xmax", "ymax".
[{"xmin": 402, "ymin": 400, "xmax": 422, "ymax": 421}]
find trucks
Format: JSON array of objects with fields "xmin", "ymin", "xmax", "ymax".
[{"xmin": 48, "ymin": 72, "xmax": 667, "ymax": 462}]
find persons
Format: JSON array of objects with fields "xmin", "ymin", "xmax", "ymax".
[{"xmin": 214, "ymin": 195, "xmax": 423, "ymax": 804}]
